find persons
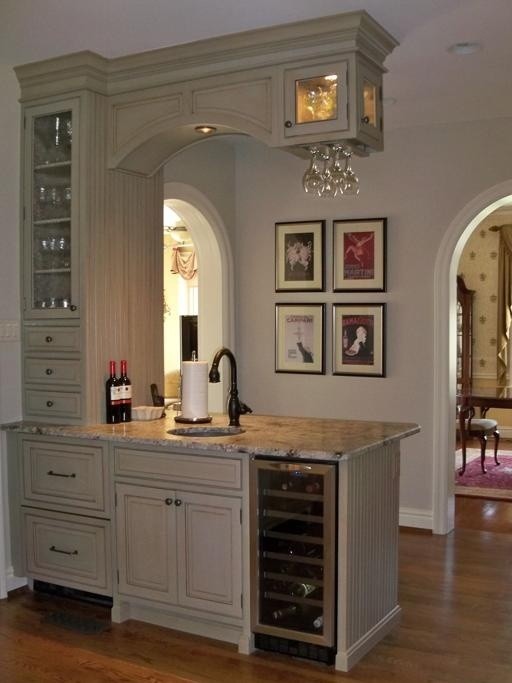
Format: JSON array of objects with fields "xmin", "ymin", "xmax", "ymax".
[
  {"xmin": 344, "ymin": 326, "xmax": 371, "ymax": 359},
  {"xmin": 344, "ymin": 232, "xmax": 373, "ymax": 268}
]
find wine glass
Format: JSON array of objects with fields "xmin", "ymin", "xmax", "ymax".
[
  {"xmin": 33, "ymin": 113, "xmax": 73, "ymax": 310},
  {"xmin": 300, "ymin": 142, "xmax": 361, "ymax": 199}
]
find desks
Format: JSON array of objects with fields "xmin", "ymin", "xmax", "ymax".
[{"xmin": 455, "ymin": 376, "xmax": 512, "ymax": 477}]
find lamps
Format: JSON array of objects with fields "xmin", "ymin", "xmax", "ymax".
[{"xmin": 162, "ymin": 223, "xmax": 192, "ymax": 245}]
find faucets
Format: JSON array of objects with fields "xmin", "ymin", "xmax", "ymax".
[{"xmin": 208, "ymin": 347, "xmax": 253, "ymax": 426}]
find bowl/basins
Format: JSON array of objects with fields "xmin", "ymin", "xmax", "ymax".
[{"xmin": 129, "ymin": 405, "xmax": 164, "ymax": 421}]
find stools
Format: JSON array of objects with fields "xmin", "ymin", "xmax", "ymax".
[{"xmin": 455, "ymin": 416, "xmax": 501, "ymax": 476}]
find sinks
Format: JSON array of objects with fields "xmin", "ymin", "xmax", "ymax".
[{"xmin": 165, "ymin": 426, "xmax": 247, "ymax": 437}]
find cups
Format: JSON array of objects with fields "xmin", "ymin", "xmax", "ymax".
[{"xmin": 301, "ymin": 81, "xmax": 337, "ymax": 120}]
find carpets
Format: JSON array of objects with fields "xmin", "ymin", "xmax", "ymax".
[{"xmin": 453, "ymin": 446, "xmax": 512, "ymax": 503}]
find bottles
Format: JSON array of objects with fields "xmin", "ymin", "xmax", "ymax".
[
  {"xmin": 105, "ymin": 360, "xmax": 120, "ymax": 424},
  {"xmin": 119, "ymin": 359, "xmax": 132, "ymax": 424}
]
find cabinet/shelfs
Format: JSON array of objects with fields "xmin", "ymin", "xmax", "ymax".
[
  {"xmin": 278, "ymin": 50, "xmax": 390, "ymax": 162},
  {"xmin": 110, "ymin": 445, "xmax": 257, "ymax": 656},
  {"xmin": 12, "ymin": 47, "xmax": 166, "ymax": 426},
  {"xmin": 7, "ymin": 429, "xmax": 115, "ymax": 596}
]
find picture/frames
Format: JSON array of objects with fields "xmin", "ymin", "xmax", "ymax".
[
  {"xmin": 272, "ymin": 217, "xmax": 327, "ymax": 294},
  {"xmin": 331, "ymin": 216, "xmax": 389, "ymax": 293},
  {"xmin": 274, "ymin": 301, "xmax": 326, "ymax": 376},
  {"xmin": 331, "ymin": 301, "xmax": 387, "ymax": 378}
]
